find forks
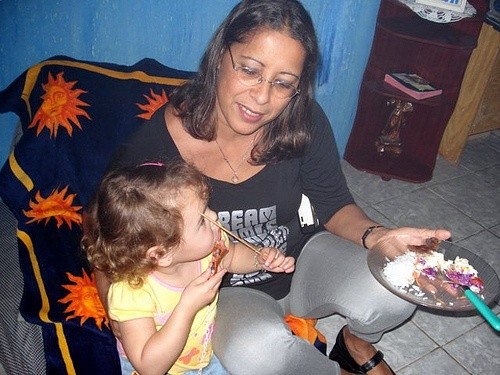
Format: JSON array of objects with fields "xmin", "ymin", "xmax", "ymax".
[{"xmin": 435, "ymin": 271, "xmax": 500, "ymax": 331}]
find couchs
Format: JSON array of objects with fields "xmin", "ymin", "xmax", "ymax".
[{"xmin": 0, "ymin": 55, "xmax": 327, "ymax": 375}]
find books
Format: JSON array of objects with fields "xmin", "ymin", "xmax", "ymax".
[{"xmin": 384, "ymin": 72, "xmax": 442, "ymax": 100}]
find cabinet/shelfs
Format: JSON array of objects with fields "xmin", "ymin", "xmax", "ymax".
[{"xmin": 344, "ymin": 0, "xmax": 490, "ymax": 184}]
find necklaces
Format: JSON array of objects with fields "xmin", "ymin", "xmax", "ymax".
[{"xmin": 207, "ymin": 121, "xmax": 267, "ymax": 183}]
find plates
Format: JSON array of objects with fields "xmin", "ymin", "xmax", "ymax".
[{"xmin": 367, "ymin": 235, "xmax": 500, "ymax": 312}]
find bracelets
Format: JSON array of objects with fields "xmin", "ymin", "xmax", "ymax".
[
  {"xmin": 253, "ymin": 248, "xmax": 264, "ymax": 272},
  {"xmin": 361, "ymin": 225, "xmax": 385, "ymax": 250}
]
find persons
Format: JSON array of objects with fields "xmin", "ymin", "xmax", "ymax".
[
  {"xmin": 94, "ymin": 0, "xmax": 452, "ymax": 375},
  {"xmin": 81, "ymin": 159, "xmax": 295, "ymax": 375}
]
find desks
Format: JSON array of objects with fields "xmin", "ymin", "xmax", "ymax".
[{"xmin": 439, "ymin": 15, "xmax": 500, "ymax": 168}]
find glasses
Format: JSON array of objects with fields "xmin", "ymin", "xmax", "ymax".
[{"xmin": 229, "ymin": 45, "xmax": 302, "ymax": 98}]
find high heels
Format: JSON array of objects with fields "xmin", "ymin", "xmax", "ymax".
[{"xmin": 327, "ymin": 324, "xmax": 399, "ymax": 375}]
